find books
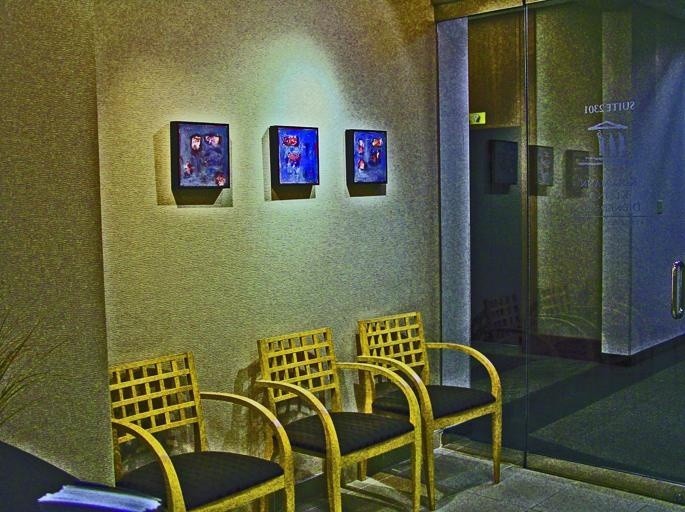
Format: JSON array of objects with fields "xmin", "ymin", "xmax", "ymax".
[{"xmin": 36, "ymin": 481, "xmax": 163, "ymax": 512}]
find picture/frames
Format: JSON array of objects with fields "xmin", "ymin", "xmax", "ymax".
[
  {"xmin": 567, "ymin": 149, "xmax": 590, "ymax": 187},
  {"xmin": 270, "ymin": 126, "xmax": 320, "ymax": 186},
  {"xmin": 488, "ymin": 139, "xmax": 518, "ymax": 186},
  {"xmin": 170, "ymin": 120, "xmax": 230, "ymax": 189},
  {"xmin": 530, "ymin": 145, "xmax": 554, "ymax": 185},
  {"xmin": 346, "ymin": 128, "xmax": 387, "ymax": 185}
]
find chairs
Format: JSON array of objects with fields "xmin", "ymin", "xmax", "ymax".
[
  {"xmin": 358, "ymin": 312, "xmax": 502, "ymax": 508},
  {"xmin": 482, "ymin": 273, "xmax": 649, "ymax": 419},
  {"xmin": 109, "ymin": 351, "xmax": 296, "ymax": 511},
  {"xmin": 256, "ymin": 327, "xmax": 422, "ymax": 512}
]
